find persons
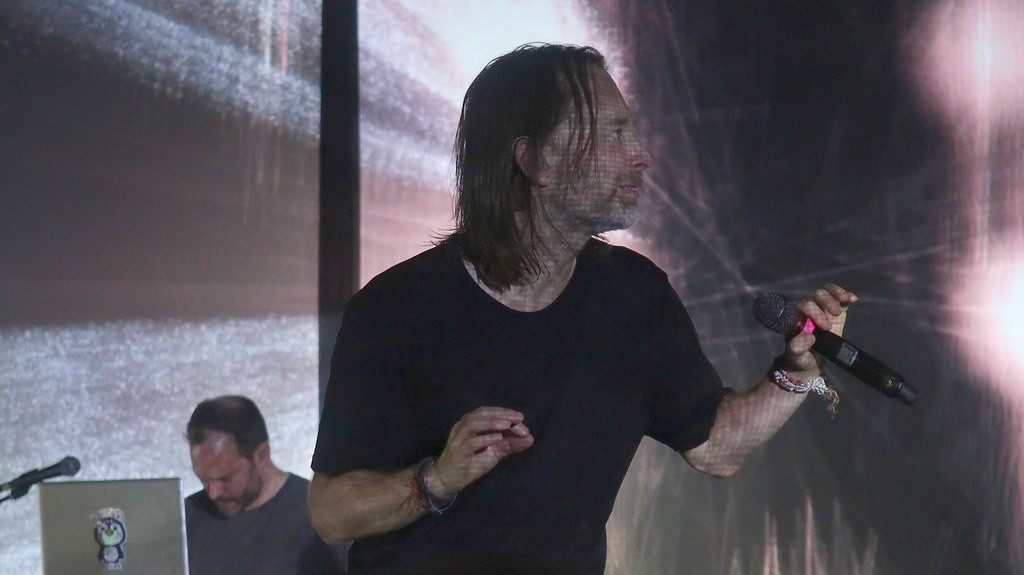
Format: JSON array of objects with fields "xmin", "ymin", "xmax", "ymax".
[
  {"xmin": 184, "ymin": 395, "xmax": 353, "ymax": 575},
  {"xmin": 306, "ymin": 45, "xmax": 858, "ymax": 575}
]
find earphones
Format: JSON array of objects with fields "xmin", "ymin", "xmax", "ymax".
[{"xmin": 534, "ymin": 155, "xmax": 549, "ymax": 172}]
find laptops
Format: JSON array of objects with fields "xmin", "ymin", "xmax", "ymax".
[{"xmin": 39, "ymin": 478, "xmax": 189, "ymax": 575}]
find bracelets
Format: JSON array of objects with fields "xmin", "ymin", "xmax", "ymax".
[
  {"xmin": 770, "ymin": 354, "xmax": 840, "ymax": 414},
  {"xmin": 410, "ymin": 456, "xmax": 458, "ymax": 516}
]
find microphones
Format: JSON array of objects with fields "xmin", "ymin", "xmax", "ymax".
[
  {"xmin": 0, "ymin": 457, "xmax": 80, "ymax": 493},
  {"xmin": 753, "ymin": 291, "xmax": 919, "ymax": 406}
]
